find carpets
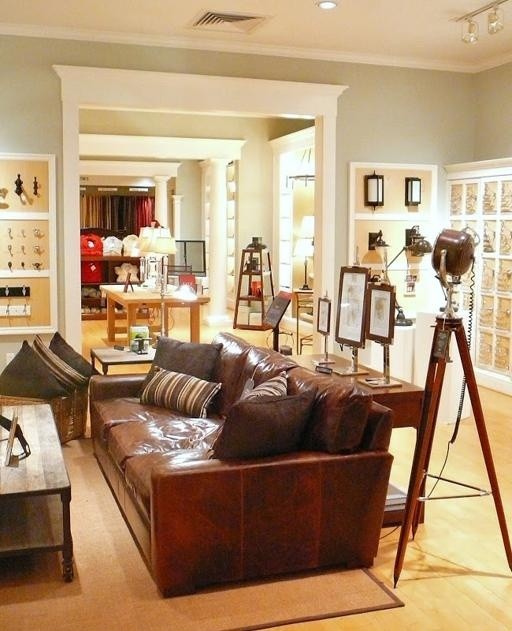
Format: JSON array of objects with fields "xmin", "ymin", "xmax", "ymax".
[{"xmin": 1, "ymin": 426, "xmax": 403, "ymax": 630}]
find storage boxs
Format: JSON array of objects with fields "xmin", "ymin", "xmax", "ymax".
[{"xmin": 0, "ymin": 385, "xmax": 88, "ymax": 444}]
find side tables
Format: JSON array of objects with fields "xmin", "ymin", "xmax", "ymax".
[{"xmin": 91, "ymin": 347, "xmax": 157, "ymax": 375}]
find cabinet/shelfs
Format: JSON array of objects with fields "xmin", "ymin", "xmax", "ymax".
[
  {"xmin": 229, "ymin": 237, "xmax": 274, "ymax": 331},
  {"xmin": 81, "ymin": 229, "xmax": 153, "ymax": 320}
]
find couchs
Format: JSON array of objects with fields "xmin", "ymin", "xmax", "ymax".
[{"xmin": 90, "ymin": 332, "xmax": 393, "ymax": 598}]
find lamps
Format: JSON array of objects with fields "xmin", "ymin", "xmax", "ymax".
[
  {"xmin": 286, "ymin": 146, "xmax": 315, "ymax": 184},
  {"xmin": 389, "ymin": 227, "xmax": 433, "ymax": 296},
  {"xmin": 405, "ymin": 177, "xmax": 421, "ymax": 206},
  {"xmin": 486, "ymin": 8, "xmax": 504, "ymax": 34},
  {"xmin": 135, "ymin": 226, "xmax": 178, "ymax": 291},
  {"xmin": 294, "ymin": 216, "xmax": 315, "ymax": 289},
  {"xmin": 462, "ymin": 19, "xmax": 479, "ymax": 44},
  {"xmin": 363, "ymin": 231, "xmax": 390, "ymax": 279},
  {"xmin": 365, "ymin": 171, "xmax": 384, "ymax": 211}
]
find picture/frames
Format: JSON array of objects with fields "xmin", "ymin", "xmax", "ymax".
[
  {"xmin": 367, "ymin": 283, "xmax": 396, "ymax": 345},
  {"xmin": 336, "ymin": 267, "xmax": 371, "ymax": 348},
  {"xmin": 318, "ymin": 299, "xmax": 330, "ymax": 335}
]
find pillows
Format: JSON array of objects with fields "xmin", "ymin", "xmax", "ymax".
[
  {"xmin": 0, "ymin": 340, "xmax": 70, "ymax": 399},
  {"xmin": 50, "ymin": 331, "xmax": 103, "ymax": 386},
  {"xmin": 205, "ymin": 388, "xmax": 317, "ymax": 461},
  {"xmin": 198, "ymin": 372, "xmax": 287, "ymax": 460},
  {"xmin": 31, "ymin": 334, "xmax": 87, "ymax": 397},
  {"xmin": 141, "ymin": 366, "xmax": 222, "ymax": 418},
  {"xmin": 141, "ymin": 336, "xmax": 223, "ymax": 396}
]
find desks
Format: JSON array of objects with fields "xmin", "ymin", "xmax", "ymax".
[
  {"xmin": 292, "ymin": 288, "xmax": 318, "ymax": 356},
  {"xmin": 284, "ymin": 352, "xmax": 424, "ymax": 527},
  {"xmin": 100, "ymin": 284, "xmax": 209, "ymax": 345},
  {"xmin": 1, "ymin": 403, "xmax": 74, "ymax": 583}
]
